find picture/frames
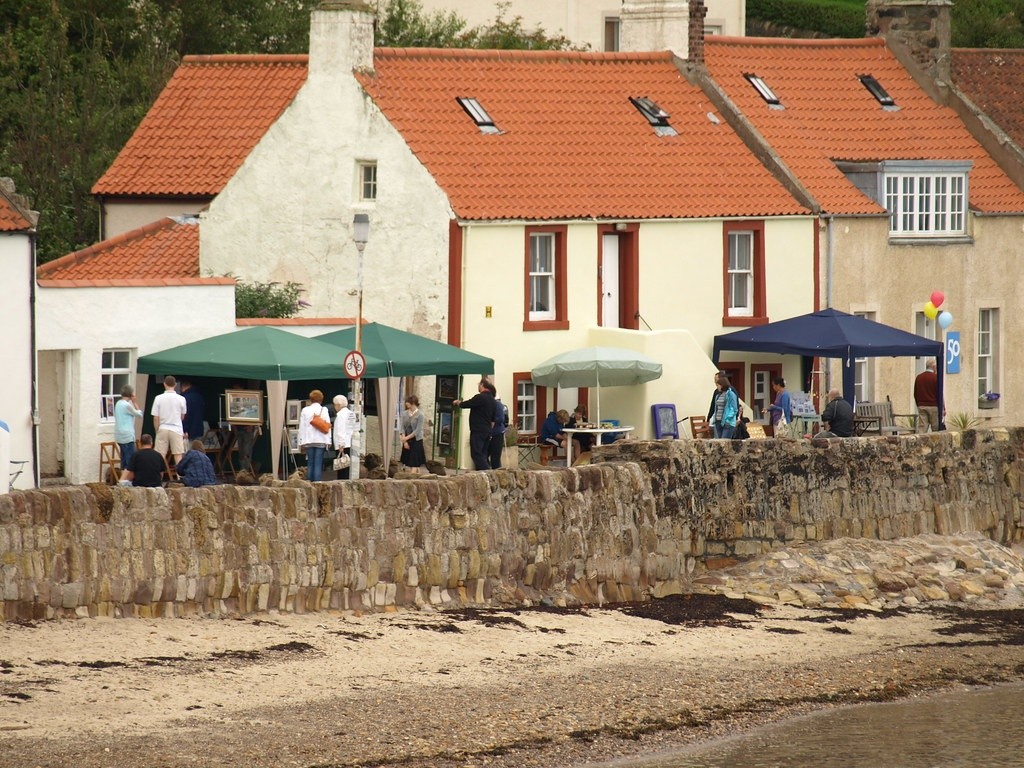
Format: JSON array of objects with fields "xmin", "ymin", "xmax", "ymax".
[
  {"xmin": 287, "ymin": 401, "xmax": 301, "ymax": 426},
  {"xmin": 435, "ymin": 375, "xmax": 460, "ymax": 403},
  {"xmin": 437, "ymin": 409, "xmax": 454, "ymax": 446},
  {"xmin": 224, "ymin": 389, "xmax": 264, "ymax": 424},
  {"xmin": 287, "ymin": 429, "xmax": 302, "ymax": 454}
]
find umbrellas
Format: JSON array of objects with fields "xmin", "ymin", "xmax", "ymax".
[{"xmin": 531, "ymin": 345, "xmax": 662, "ymax": 445}]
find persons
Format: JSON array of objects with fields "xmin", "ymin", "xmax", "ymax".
[
  {"xmin": 705, "ymin": 372, "xmax": 750, "ymax": 440},
  {"xmin": 175, "ymin": 440, "xmax": 217, "ymax": 486},
  {"xmin": 179, "ymin": 378, "xmax": 207, "ymax": 452},
  {"xmin": 150, "ymin": 376, "xmax": 186, "ymax": 481},
  {"xmin": 564, "ymin": 405, "xmax": 596, "ymax": 452},
  {"xmin": 332, "ymin": 394, "xmax": 355, "ymax": 480},
  {"xmin": 297, "ymin": 389, "xmax": 332, "ymax": 481},
  {"xmin": 541, "ymin": 410, "xmax": 580, "ymax": 464},
  {"xmin": 453, "ymin": 378, "xmax": 496, "ymax": 470},
  {"xmin": 487, "ymin": 388, "xmax": 505, "ymax": 469},
  {"xmin": 913, "ymin": 358, "xmax": 947, "ymax": 433},
  {"xmin": 115, "ymin": 385, "xmax": 142, "ymax": 486},
  {"xmin": 814, "ymin": 389, "xmax": 853, "ymax": 439},
  {"xmin": 127, "ymin": 434, "xmax": 166, "ymax": 488},
  {"xmin": 400, "ymin": 396, "xmax": 426, "ymax": 473},
  {"xmin": 761, "ymin": 377, "xmax": 791, "ymax": 439}
]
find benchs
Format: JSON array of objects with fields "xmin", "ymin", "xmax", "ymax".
[{"xmin": 855, "ymin": 395, "xmax": 918, "ymax": 435}]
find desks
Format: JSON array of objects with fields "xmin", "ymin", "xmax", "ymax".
[
  {"xmin": 853, "ymin": 419, "xmax": 876, "ymax": 437},
  {"xmin": 561, "ymin": 427, "xmax": 635, "ymax": 467}
]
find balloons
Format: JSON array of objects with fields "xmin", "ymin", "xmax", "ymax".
[
  {"xmin": 930, "ymin": 290, "xmax": 944, "ymax": 308},
  {"xmin": 924, "ymin": 302, "xmax": 937, "ymax": 321},
  {"xmin": 938, "ymin": 312, "xmax": 952, "ymax": 329}
]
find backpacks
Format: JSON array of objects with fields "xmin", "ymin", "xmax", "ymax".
[{"xmin": 495, "ymin": 399, "xmax": 509, "ymax": 427}]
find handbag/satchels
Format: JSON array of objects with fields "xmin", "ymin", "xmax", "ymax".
[
  {"xmin": 941, "ymin": 416, "xmax": 946, "ymax": 431},
  {"xmin": 776, "ymin": 408, "xmax": 790, "ymax": 438},
  {"xmin": 332, "ymin": 451, "xmax": 351, "ymax": 471},
  {"xmin": 309, "ymin": 407, "xmax": 332, "ymax": 434}
]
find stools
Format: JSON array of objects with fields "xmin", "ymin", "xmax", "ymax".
[
  {"xmin": 515, "ymin": 443, "xmax": 535, "ymax": 464},
  {"xmin": 537, "ymin": 444, "xmax": 554, "ymax": 465}
]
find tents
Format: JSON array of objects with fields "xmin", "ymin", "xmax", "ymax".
[
  {"xmin": 137, "ymin": 326, "xmax": 394, "ymax": 480},
  {"xmin": 711, "ymin": 307, "xmax": 944, "ymax": 431},
  {"xmin": 309, "ymin": 322, "xmax": 495, "ymax": 479}
]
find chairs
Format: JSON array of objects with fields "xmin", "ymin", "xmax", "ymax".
[
  {"xmin": 201, "ymin": 429, "xmax": 229, "ymax": 481},
  {"xmin": 690, "ymin": 416, "xmax": 714, "ymax": 439},
  {"xmin": 224, "ymin": 429, "xmax": 257, "ymax": 480}
]
